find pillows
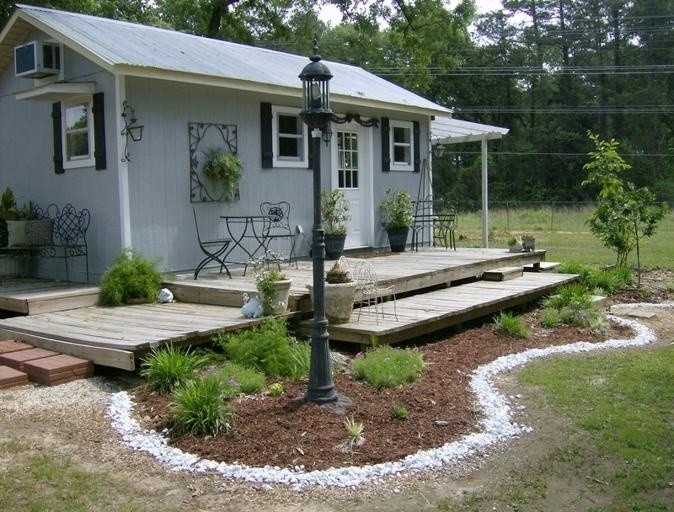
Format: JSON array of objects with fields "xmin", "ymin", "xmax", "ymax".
[{"xmin": 3, "ymin": 219, "xmax": 54, "ymax": 249}]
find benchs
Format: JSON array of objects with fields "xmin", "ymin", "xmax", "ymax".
[{"xmin": 0, "ymin": 202, "xmax": 91, "ymax": 286}]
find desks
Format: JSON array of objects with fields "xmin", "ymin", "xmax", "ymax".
[{"xmin": 220, "ymin": 215, "xmax": 282, "ymax": 277}]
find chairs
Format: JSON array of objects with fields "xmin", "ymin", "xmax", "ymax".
[
  {"xmin": 259, "ymin": 201, "xmax": 298, "ymax": 272},
  {"xmin": 338, "ymin": 255, "xmax": 400, "ymax": 326},
  {"xmin": 409, "ymin": 195, "xmax": 459, "ymax": 253},
  {"xmin": 192, "ymin": 207, "xmax": 233, "ymax": 280}
]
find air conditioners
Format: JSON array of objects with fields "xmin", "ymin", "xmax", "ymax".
[{"xmin": 13, "ymin": 39, "xmax": 61, "ymax": 79}]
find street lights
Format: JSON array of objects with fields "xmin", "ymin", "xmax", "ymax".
[{"xmin": 299, "ymin": 29, "xmax": 341, "ymax": 403}]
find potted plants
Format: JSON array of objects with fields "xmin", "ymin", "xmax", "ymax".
[
  {"xmin": 253, "ymin": 270, "xmax": 291, "ymax": 315},
  {"xmin": 321, "ymin": 188, "xmax": 353, "ymax": 259},
  {"xmin": 507, "ymin": 236, "xmax": 522, "ymax": 251},
  {"xmin": 378, "ymin": 187, "xmax": 413, "ymax": 252},
  {"xmin": 305, "ymin": 260, "xmax": 358, "ymax": 324}
]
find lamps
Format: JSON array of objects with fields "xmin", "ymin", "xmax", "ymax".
[
  {"xmin": 429, "ymin": 131, "xmax": 446, "ymax": 158},
  {"xmin": 321, "ymin": 123, "xmax": 332, "ymax": 148},
  {"xmin": 120, "ymin": 99, "xmax": 144, "ymax": 164}
]
